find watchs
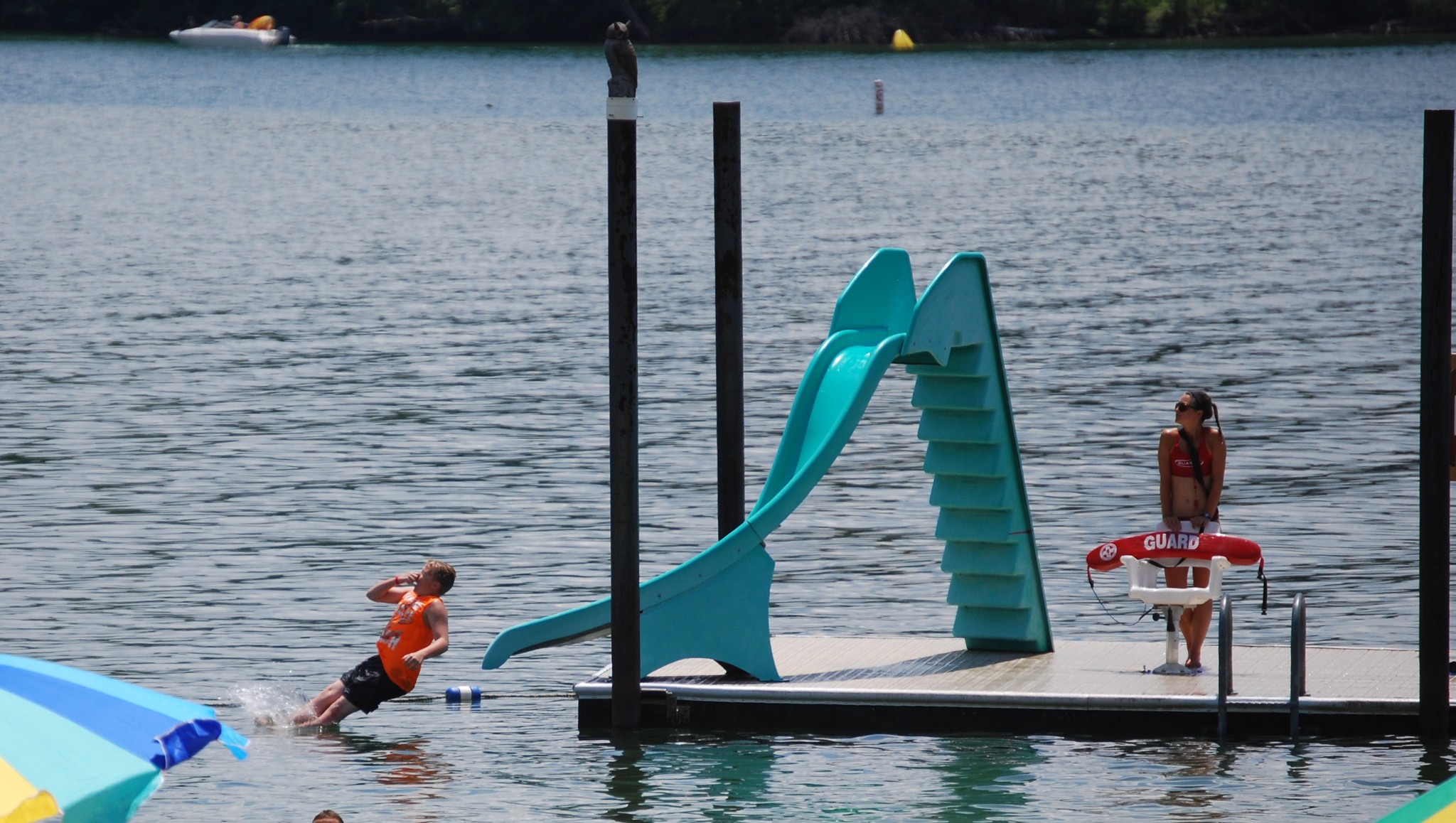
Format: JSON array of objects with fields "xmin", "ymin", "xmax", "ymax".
[{"xmin": 1204, "ymin": 513, "xmax": 1213, "ymax": 521}]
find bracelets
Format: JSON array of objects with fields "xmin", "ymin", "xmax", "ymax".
[{"xmin": 395, "ymin": 576, "xmax": 400, "ymax": 585}]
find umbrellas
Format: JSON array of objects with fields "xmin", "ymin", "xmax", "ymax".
[{"xmin": 0, "ymin": 653, "xmax": 253, "ymax": 823}]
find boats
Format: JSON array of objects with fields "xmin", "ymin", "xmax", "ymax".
[{"xmin": 169, "ymin": 14, "xmax": 298, "ymax": 46}]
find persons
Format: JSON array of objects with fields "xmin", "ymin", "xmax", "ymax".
[
  {"xmin": 1158, "ymin": 390, "xmax": 1226, "ymax": 668},
  {"xmin": 312, "ymin": 810, "xmax": 344, "ymax": 823},
  {"xmin": 253, "ymin": 559, "xmax": 457, "ymax": 730},
  {"xmin": 232, "ymin": 15, "xmax": 244, "ymax": 28}
]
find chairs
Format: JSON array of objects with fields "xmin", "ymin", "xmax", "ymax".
[{"xmin": 1120, "ymin": 521, "xmax": 1231, "ymax": 675}]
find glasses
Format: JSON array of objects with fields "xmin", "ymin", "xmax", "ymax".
[{"xmin": 1175, "ymin": 402, "xmax": 1198, "ymax": 412}]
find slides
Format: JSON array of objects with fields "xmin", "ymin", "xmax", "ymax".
[{"xmin": 475, "ymin": 316, "xmax": 900, "ymax": 669}]
